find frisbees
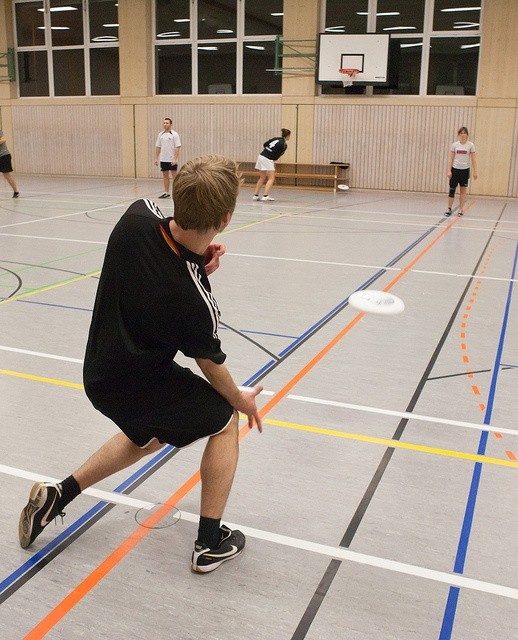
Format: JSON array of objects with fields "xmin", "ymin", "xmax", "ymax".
[
  {"xmin": 349, "ymin": 290, "xmax": 404, "ymax": 316},
  {"xmin": 337, "ymin": 184, "xmax": 349, "ymax": 190}
]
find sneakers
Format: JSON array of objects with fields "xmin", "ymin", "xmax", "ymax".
[
  {"xmin": 445, "ymin": 208, "xmax": 452, "ymax": 217},
  {"xmin": 253, "ymin": 196, "xmax": 262, "ymax": 200},
  {"xmin": 192, "ymin": 525, "xmax": 245, "ymax": 573},
  {"xmin": 13, "ymin": 192, "xmax": 19, "ymax": 198},
  {"xmin": 458, "ymin": 210, "xmax": 463, "ymax": 216},
  {"xmin": 19, "ymin": 482, "xmax": 66, "ymax": 549},
  {"xmin": 263, "ymin": 196, "xmax": 274, "ymax": 200},
  {"xmin": 158, "ymin": 193, "xmax": 170, "ymax": 199}
]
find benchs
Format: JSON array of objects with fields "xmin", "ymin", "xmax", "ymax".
[{"xmin": 235, "ymin": 160, "xmax": 350, "ymax": 195}]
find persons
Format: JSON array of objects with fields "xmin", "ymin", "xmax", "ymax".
[
  {"xmin": 252, "ymin": 129, "xmax": 291, "ymax": 202},
  {"xmin": 19, "ymin": 154, "xmax": 262, "ymax": 573},
  {"xmin": 2, "ymin": 135, "xmax": 20, "ymax": 198},
  {"xmin": 155, "ymin": 118, "xmax": 180, "ymax": 199},
  {"xmin": 445, "ymin": 126, "xmax": 477, "ymax": 217}
]
